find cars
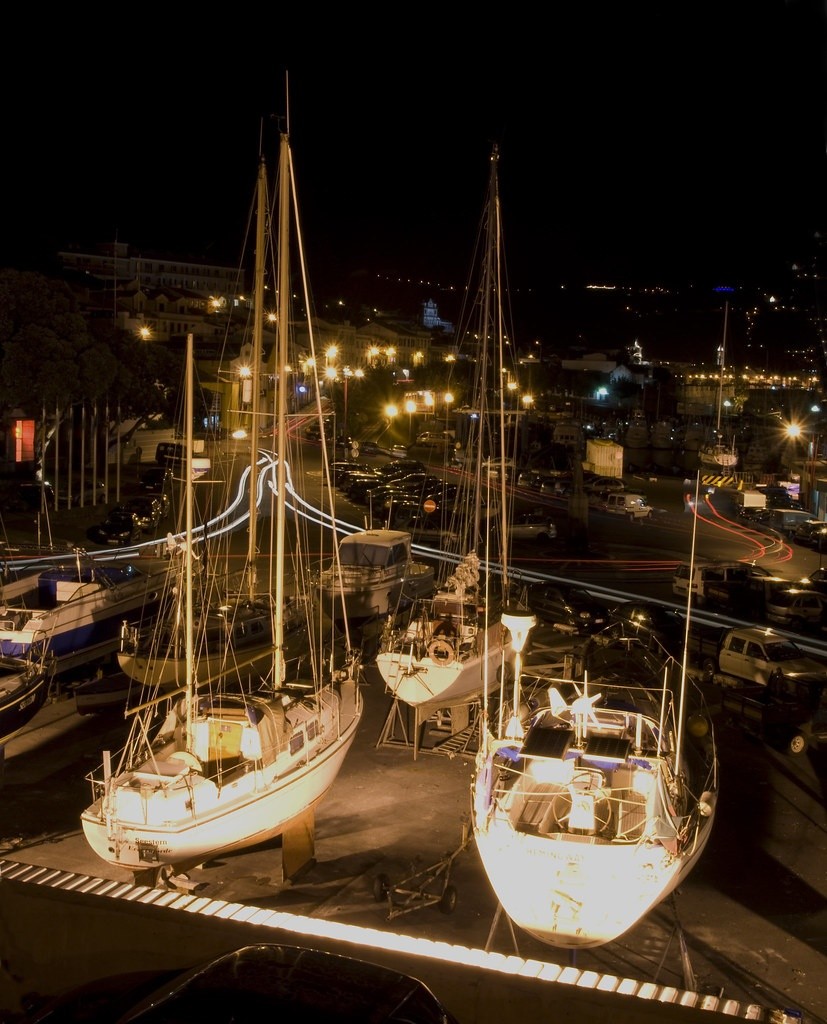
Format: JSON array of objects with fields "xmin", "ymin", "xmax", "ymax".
[
  {"xmin": 98, "ymin": 492, "xmax": 170, "ymax": 545},
  {"xmin": 528, "ymin": 580, "xmax": 693, "ymax": 659},
  {"xmin": 137, "ymin": 466, "xmax": 174, "ymax": 494},
  {"xmin": 389, "ymin": 444, "xmax": 408, "ymax": 458},
  {"xmin": 739, "ymin": 485, "xmax": 827, "ymax": 552},
  {"xmin": 478, "ymin": 456, "xmax": 653, "ymax": 522},
  {"xmin": 415, "ymin": 431, "xmax": 455, "ymax": 450},
  {"xmin": 766, "ymin": 590, "xmax": 827, "ymax": 633}
]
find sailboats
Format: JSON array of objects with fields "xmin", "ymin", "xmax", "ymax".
[{"xmin": 79, "ymin": 52, "xmax": 371, "ymax": 870}]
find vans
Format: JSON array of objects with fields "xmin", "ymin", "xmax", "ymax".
[{"xmin": 672, "ymin": 562, "xmax": 754, "ymax": 604}]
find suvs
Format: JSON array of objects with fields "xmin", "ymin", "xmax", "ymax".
[{"xmin": 323, "ymin": 459, "xmax": 559, "ymax": 551}]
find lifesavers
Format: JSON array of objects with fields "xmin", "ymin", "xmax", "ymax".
[{"xmin": 428, "ymin": 639, "xmax": 454, "ymax": 667}]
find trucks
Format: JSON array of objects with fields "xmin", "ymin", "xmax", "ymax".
[
  {"xmin": 703, "ymin": 570, "xmax": 792, "ymax": 613},
  {"xmin": 683, "ymin": 626, "xmax": 826, "ymax": 709}
]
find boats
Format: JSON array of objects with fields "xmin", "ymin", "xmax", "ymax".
[
  {"xmin": 376, "ymin": 131, "xmax": 543, "ymax": 710},
  {"xmin": 623, "ymin": 301, "xmax": 779, "ymax": 481},
  {"xmin": 548, "ymin": 419, "xmax": 586, "ymax": 452},
  {"xmin": 305, "ymin": 490, "xmax": 436, "ymax": 634},
  {"xmin": 1, "ymin": 548, "xmax": 206, "ymax": 680},
  {"xmin": 469, "ymin": 467, "xmax": 721, "ymax": 952},
  {"xmin": 0, "ymin": 604, "xmax": 60, "ymax": 738}
]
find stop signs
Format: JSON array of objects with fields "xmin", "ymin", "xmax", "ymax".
[{"xmin": 424, "ymin": 499, "xmax": 436, "ymax": 513}]
